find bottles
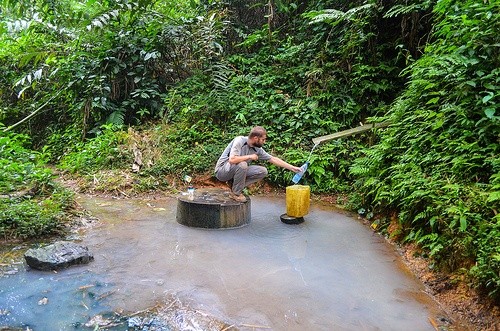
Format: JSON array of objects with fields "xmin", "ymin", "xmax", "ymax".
[
  {"xmin": 188, "ymin": 183, "xmax": 194, "ymax": 201},
  {"xmin": 292, "ymin": 162, "xmax": 308, "ymax": 184}
]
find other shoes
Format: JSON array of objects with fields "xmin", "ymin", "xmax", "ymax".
[
  {"xmin": 229, "ymin": 192, "xmax": 247, "ymax": 203},
  {"xmin": 226, "ymin": 180, "xmax": 245, "ymax": 193}
]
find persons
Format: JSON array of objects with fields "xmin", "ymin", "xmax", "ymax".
[{"xmin": 215, "ymin": 126, "xmax": 302, "ymax": 203}]
